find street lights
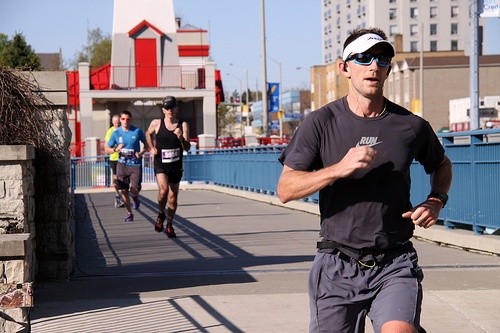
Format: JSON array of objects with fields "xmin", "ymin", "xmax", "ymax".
[
  {"xmin": 297, "ymin": 67, "xmax": 321, "ymax": 109},
  {"xmin": 229, "ymin": 63, "xmax": 249, "ymax": 126},
  {"xmin": 260, "ymin": 55, "xmax": 283, "ymax": 138},
  {"xmin": 226, "ymin": 73, "xmax": 242, "ymax": 124}
]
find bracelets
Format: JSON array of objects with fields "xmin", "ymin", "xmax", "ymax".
[
  {"xmin": 180, "ymin": 138, "xmax": 185, "ymax": 143},
  {"xmin": 114, "ymin": 147, "xmax": 117, "ymax": 152}
]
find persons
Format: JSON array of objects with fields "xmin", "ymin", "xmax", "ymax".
[
  {"xmin": 276, "ymin": 29, "xmax": 452, "ymax": 333},
  {"xmin": 105, "ymin": 111, "xmax": 149, "ymax": 222},
  {"xmin": 145, "ymin": 96, "xmax": 190, "ymax": 238},
  {"xmin": 104, "ymin": 115, "xmax": 129, "ymax": 208}
]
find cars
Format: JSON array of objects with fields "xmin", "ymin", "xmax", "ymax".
[{"xmin": 268, "ymin": 121, "xmax": 279, "ymax": 130}]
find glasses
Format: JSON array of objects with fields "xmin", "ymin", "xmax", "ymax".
[
  {"xmin": 345, "ymin": 51, "xmax": 392, "ymax": 66},
  {"xmin": 163, "ymin": 102, "xmax": 175, "ymax": 109},
  {"xmin": 121, "ymin": 117, "xmax": 130, "ymax": 121}
]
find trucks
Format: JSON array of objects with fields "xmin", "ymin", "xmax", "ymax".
[{"xmin": 449, "ymin": 96, "xmax": 500, "ymax": 130}]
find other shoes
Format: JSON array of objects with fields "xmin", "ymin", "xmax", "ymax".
[
  {"xmin": 125, "ymin": 214, "xmax": 134, "ymax": 222},
  {"xmin": 133, "ymin": 197, "xmax": 140, "ymax": 210},
  {"xmin": 115, "ymin": 194, "xmax": 125, "ymax": 208}
]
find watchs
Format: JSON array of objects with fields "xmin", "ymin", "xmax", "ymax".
[{"xmin": 427, "ymin": 191, "xmax": 448, "ymax": 208}]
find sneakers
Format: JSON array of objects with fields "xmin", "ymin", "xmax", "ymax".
[
  {"xmin": 166, "ymin": 224, "xmax": 176, "ymax": 238},
  {"xmin": 155, "ymin": 214, "xmax": 166, "ymax": 232}
]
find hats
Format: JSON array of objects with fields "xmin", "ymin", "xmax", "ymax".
[
  {"xmin": 343, "ymin": 33, "xmax": 395, "ymax": 62},
  {"xmin": 163, "ymin": 96, "xmax": 176, "ymax": 106}
]
